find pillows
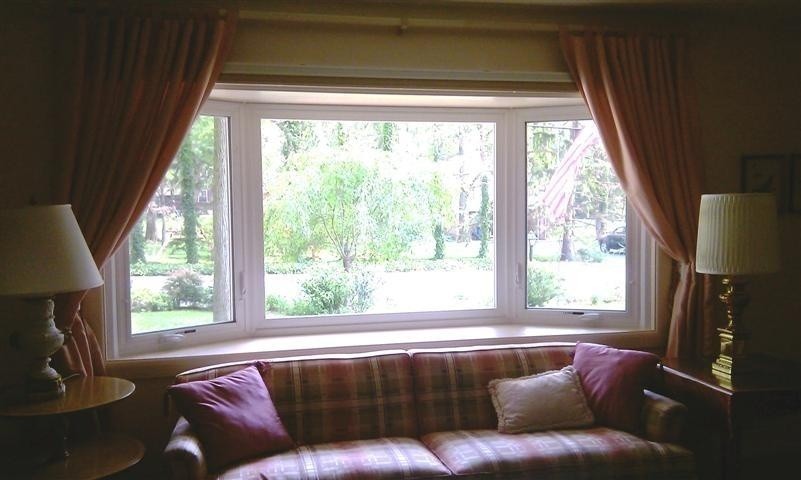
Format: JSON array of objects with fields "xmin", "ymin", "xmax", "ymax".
[
  {"xmin": 168, "ymin": 364, "xmax": 296, "ymax": 473},
  {"xmin": 488, "ymin": 339, "xmax": 660, "ymax": 436}
]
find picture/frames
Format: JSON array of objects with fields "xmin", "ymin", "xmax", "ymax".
[{"xmin": 740, "ymin": 154, "xmax": 795, "ymax": 214}]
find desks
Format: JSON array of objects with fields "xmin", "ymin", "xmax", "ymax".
[
  {"xmin": 654, "ymin": 355, "xmax": 799, "ymax": 480},
  {"xmin": 2, "ymin": 377, "xmax": 146, "ymax": 479}
]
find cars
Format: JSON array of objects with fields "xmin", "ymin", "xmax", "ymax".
[{"xmin": 600, "ymin": 226, "xmax": 629, "ymax": 254}]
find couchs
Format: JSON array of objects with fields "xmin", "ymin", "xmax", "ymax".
[{"xmin": 162, "ymin": 342, "xmax": 697, "ymax": 478}]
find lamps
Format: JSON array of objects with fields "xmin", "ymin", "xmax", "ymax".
[
  {"xmin": 696, "ymin": 192, "xmax": 783, "ymax": 385},
  {"xmin": 0, "ymin": 202, "xmax": 104, "ymax": 403}
]
